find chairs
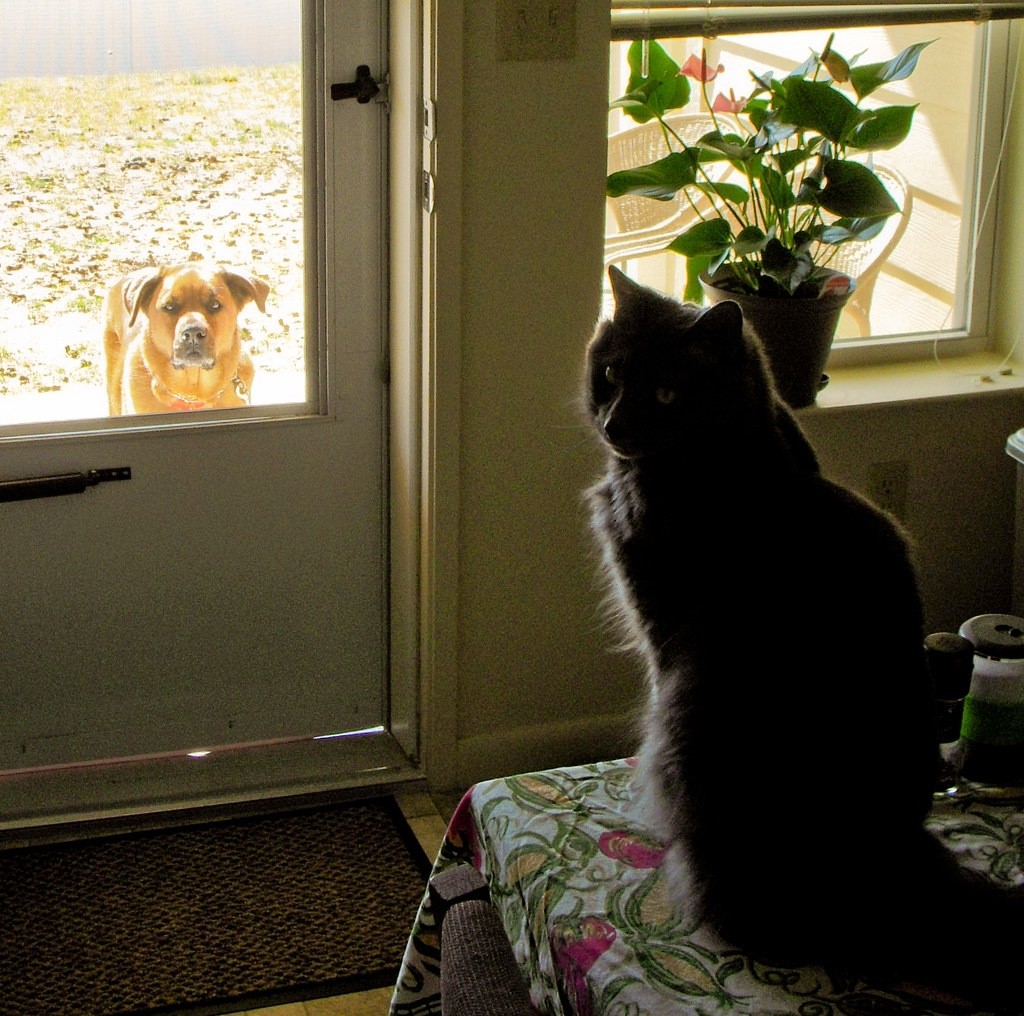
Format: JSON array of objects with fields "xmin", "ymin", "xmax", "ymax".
[
  {"xmin": 602, "ymin": 111, "xmax": 749, "ymax": 297},
  {"xmin": 603, "ymin": 161, "xmax": 913, "ymax": 339}
]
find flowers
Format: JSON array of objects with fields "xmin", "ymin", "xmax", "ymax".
[{"xmin": 605, "ymin": 39, "xmax": 940, "ymax": 303}]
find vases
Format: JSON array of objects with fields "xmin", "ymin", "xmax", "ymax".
[{"xmin": 694, "ymin": 262, "xmax": 855, "ymax": 409}]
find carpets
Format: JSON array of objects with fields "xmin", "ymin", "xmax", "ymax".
[{"xmin": 0, "ymin": 795, "xmax": 434, "ymax": 1016}]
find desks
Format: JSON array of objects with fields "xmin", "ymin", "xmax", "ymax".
[{"xmin": 386, "ymin": 755, "xmax": 1024, "ymax": 1016}]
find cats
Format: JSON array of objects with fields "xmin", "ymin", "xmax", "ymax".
[{"xmin": 583, "ymin": 262, "xmax": 1024, "ymax": 1016}]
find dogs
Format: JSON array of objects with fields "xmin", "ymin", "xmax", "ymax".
[{"xmin": 103, "ymin": 264, "xmax": 271, "ymax": 415}]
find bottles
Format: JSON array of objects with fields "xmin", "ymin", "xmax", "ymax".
[
  {"xmin": 922, "ymin": 632, "xmax": 976, "ymax": 801},
  {"xmin": 957, "ymin": 613, "xmax": 1024, "ymax": 785}
]
